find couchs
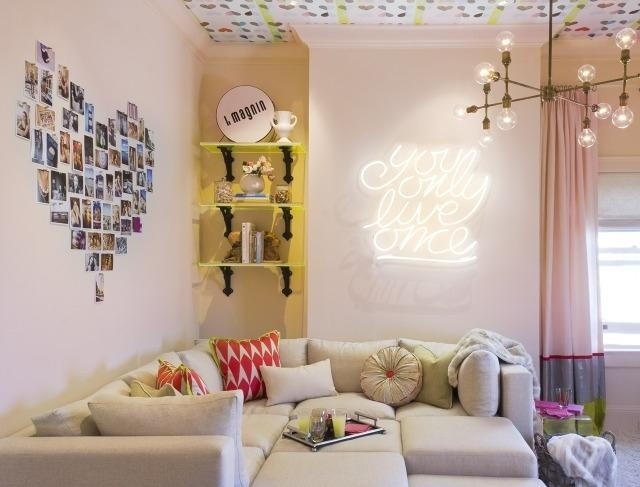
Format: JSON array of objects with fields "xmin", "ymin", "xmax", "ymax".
[{"xmin": 1, "ymin": 338, "xmax": 534, "ymax": 486}]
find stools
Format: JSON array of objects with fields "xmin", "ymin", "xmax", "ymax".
[{"xmin": 251, "ymin": 414, "xmax": 547, "ymax": 486}]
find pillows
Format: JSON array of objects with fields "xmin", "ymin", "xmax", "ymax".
[
  {"xmin": 458, "ymin": 351, "xmax": 500, "ymax": 417},
  {"xmin": 260, "ymin": 362, "xmax": 336, "ymax": 403},
  {"xmin": 412, "ymin": 345, "xmax": 455, "ymax": 408},
  {"xmin": 360, "ymin": 346, "xmax": 423, "ymax": 406},
  {"xmin": 31, "ymin": 328, "xmax": 281, "ymax": 485},
  {"xmin": 305, "ymin": 338, "xmax": 400, "ymax": 395}
]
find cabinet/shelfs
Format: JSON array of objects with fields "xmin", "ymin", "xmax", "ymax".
[{"xmin": 198, "ymin": 136, "xmax": 296, "ymax": 298}]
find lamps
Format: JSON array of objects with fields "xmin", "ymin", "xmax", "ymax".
[{"xmin": 452, "ymin": 1, "xmax": 639, "ymax": 149}]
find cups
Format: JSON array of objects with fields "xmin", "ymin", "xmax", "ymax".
[
  {"xmin": 332, "ymin": 407, "xmax": 346, "ymax": 437},
  {"xmin": 275, "ymin": 185, "xmax": 290, "ymax": 202},
  {"xmin": 296, "ymin": 406, "xmax": 327, "ymax": 442}
]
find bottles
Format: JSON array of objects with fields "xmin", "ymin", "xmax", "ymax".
[{"xmin": 213, "ymin": 180, "xmax": 233, "ymax": 203}]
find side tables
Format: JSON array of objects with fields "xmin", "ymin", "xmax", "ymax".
[{"xmin": 536, "ymin": 414, "xmax": 593, "ymax": 437}]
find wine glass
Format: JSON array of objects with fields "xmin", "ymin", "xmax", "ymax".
[{"xmin": 554, "ymin": 383, "xmax": 574, "ymax": 419}]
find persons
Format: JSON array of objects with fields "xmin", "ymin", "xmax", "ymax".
[{"xmin": 17, "ymin": 43, "xmax": 154, "ymax": 303}]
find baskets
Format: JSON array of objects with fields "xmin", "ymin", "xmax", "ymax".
[{"xmin": 535, "ymin": 431, "xmax": 615, "ymax": 487}]
